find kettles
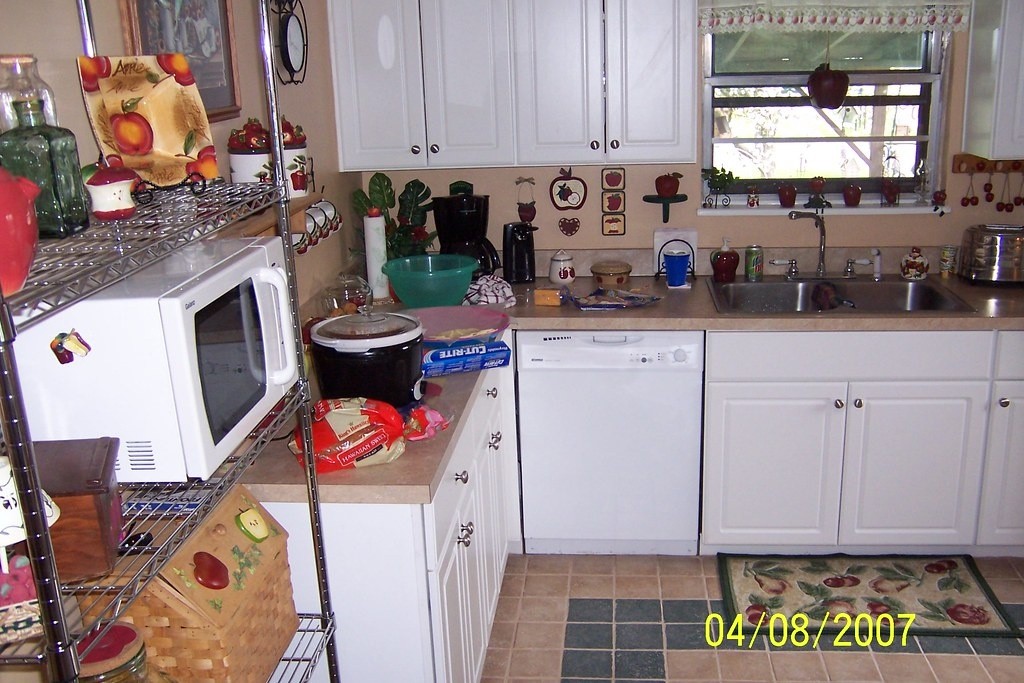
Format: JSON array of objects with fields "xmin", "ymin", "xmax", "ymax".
[{"xmin": 502, "ymin": 223, "xmax": 539, "ymax": 283}]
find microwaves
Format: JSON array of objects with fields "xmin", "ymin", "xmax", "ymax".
[{"xmin": 4, "ymin": 236, "xmax": 299, "ymax": 481}]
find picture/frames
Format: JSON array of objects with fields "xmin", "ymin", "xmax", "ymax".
[{"xmin": 117, "ymin": 0, "xmax": 243, "ymax": 124}]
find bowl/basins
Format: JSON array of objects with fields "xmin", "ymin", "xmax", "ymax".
[
  {"xmin": 227, "ymin": 141, "xmax": 310, "ymax": 200},
  {"xmin": 958, "ymin": 224, "xmax": 1023, "ymax": 282},
  {"xmin": 381, "ymin": 256, "xmax": 481, "ymax": 307},
  {"xmin": 393, "ymin": 305, "xmax": 509, "ymax": 342},
  {"xmin": 319, "ymin": 275, "xmax": 373, "ymax": 316},
  {"xmin": 590, "ymin": 261, "xmax": 632, "ymax": 290}
]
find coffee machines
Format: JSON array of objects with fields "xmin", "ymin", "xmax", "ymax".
[{"xmin": 431, "ymin": 195, "xmax": 501, "ymax": 281}]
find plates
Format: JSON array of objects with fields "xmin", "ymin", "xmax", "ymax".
[{"xmin": 77, "ymin": 52, "xmax": 219, "ymax": 188}]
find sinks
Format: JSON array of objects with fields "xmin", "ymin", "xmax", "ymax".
[
  {"xmin": 834, "ymin": 274, "xmax": 978, "ymax": 315},
  {"xmin": 705, "ymin": 274, "xmax": 846, "ymax": 318}
]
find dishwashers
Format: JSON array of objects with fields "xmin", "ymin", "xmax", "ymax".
[{"xmin": 515, "ymin": 331, "xmax": 699, "ymax": 556}]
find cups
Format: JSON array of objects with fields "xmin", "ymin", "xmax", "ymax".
[
  {"xmin": 663, "ymin": 250, "xmax": 689, "ymax": 286},
  {"xmin": 291, "ymin": 198, "xmax": 344, "ymax": 255},
  {"xmin": 550, "ymin": 248, "xmax": 576, "ymax": 283}
]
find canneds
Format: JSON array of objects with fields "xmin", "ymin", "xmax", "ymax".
[{"xmin": 745, "ymin": 243, "xmax": 764, "ymax": 282}]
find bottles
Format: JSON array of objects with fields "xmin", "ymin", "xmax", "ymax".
[
  {"xmin": 0, "ymin": 98, "xmax": 90, "ymax": 238},
  {"xmin": 70, "ymin": 622, "xmax": 177, "ymax": 683},
  {"xmin": 843, "ymin": 185, "xmax": 861, "ymax": 206},
  {"xmin": 747, "ymin": 185, "xmax": 760, "ymax": 208},
  {"xmin": 0, "ymin": 54, "xmax": 59, "ymax": 134},
  {"xmin": 778, "ymin": 184, "xmax": 796, "ymax": 207},
  {"xmin": 900, "ymin": 246, "xmax": 929, "ymax": 280}
]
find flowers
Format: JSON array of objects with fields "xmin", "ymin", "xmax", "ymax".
[{"xmin": 346, "ymin": 173, "xmax": 438, "ymax": 261}]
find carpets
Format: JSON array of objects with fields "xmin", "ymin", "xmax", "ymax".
[{"xmin": 716, "ymin": 552, "xmax": 1024, "ymax": 639}]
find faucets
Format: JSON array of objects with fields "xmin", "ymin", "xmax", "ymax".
[{"xmin": 788, "ymin": 210, "xmax": 826, "ymax": 274}]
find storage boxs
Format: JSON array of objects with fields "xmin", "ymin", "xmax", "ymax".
[{"xmin": 421, "ymin": 340, "xmax": 512, "ymax": 379}]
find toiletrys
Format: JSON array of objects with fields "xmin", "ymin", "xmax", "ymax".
[{"xmin": 710, "ymin": 236, "xmax": 740, "ymax": 283}]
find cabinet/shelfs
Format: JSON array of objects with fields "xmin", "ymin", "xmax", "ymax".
[
  {"xmin": 960, "ymin": 0, "xmax": 1024, "ymax": 161},
  {"xmin": 325, "ymin": 0, "xmax": 516, "ymax": 173},
  {"xmin": 0, "ymin": 0, "xmax": 341, "ymax": 683},
  {"xmin": 971, "ymin": 330, "xmax": 1024, "ymax": 558},
  {"xmin": 697, "ymin": 329, "xmax": 999, "ymax": 555},
  {"xmin": 470, "ymin": 336, "xmax": 516, "ymax": 630},
  {"xmin": 511, "ymin": 0, "xmax": 701, "ymax": 168},
  {"xmin": 259, "ymin": 424, "xmax": 490, "ymax": 683}
]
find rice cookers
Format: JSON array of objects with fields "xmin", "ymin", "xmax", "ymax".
[{"xmin": 310, "ymin": 311, "xmax": 427, "ymax": 407}]
